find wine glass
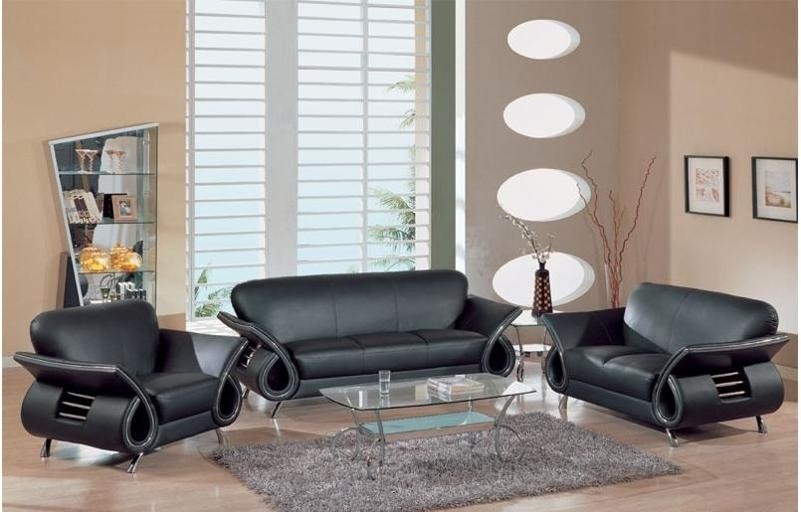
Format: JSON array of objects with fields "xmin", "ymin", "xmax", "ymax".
[{"xmin": 75, "ymin": 148, "xmax": 126, "ymax": 174}]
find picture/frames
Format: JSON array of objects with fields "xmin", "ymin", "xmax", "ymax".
[
  {"xmin": 752, "ymin": 156, "xmax": 797, "ymax": 227},
  {"xmin": 682, "ymin": 154, "xmax": 729, "ymax": 219},
  {"xmin": 111, "ymin": 195, "xmax": 137, "ymax": 222}
]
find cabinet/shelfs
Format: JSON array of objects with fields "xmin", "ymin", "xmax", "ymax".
[{"xmin": 45, "ymin": 124, "xmax": 157, "ymax": 309}]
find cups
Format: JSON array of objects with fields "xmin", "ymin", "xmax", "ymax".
[
  {"xmin": 379, "ymin": 369, "xmax": 391, "ymax": 395},
  {"xmin": 379, "ymin": 396, "xmax": 390, "ymax": 408},
  {"xmin": 100, "ymin": 287, "xmax": 111, "ymax": 303}
]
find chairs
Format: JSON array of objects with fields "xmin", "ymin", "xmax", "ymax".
[
  {"xmin": 11, "ymin": 297, "xmax": 225, "ymax": 471},
  {"xmin": 541, "ymin": 281, "xmax": 789, "ymax": 448}
]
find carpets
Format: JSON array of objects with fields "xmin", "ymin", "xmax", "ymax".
[{"xmin": 196, "ymin": 410, "xmax": 687, "ymax": 512}]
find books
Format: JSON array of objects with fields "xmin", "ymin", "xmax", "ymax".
[{"xmin": 428, "ymin": 376, "xmax": 485, "ymax": 394}]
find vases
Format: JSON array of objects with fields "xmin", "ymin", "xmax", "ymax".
[
  {"xmin": 533, "ymin": 262, "xmax": 553, "ymax": 318},
  {"xmin": 606, "ymin": 261, "xmax": 623, "ymax": 309}
]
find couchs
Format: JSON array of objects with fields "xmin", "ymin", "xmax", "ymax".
[{"xmin": 217, "ymin": 269, "xmax": 523, "ymax": 422}]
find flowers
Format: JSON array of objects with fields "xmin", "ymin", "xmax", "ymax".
[{"xmin": 495, "ymin": 203, "xmax": 555, "ymax": 261}]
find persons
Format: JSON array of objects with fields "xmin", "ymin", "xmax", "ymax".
[{"xmin": 120, "ymin": 201, "xmax": 131, "ymax": 215}]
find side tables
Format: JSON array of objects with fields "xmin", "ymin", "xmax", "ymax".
[{"xmin": 509, "ymin": 309, "xmax": 564, "ymax": 403}]
